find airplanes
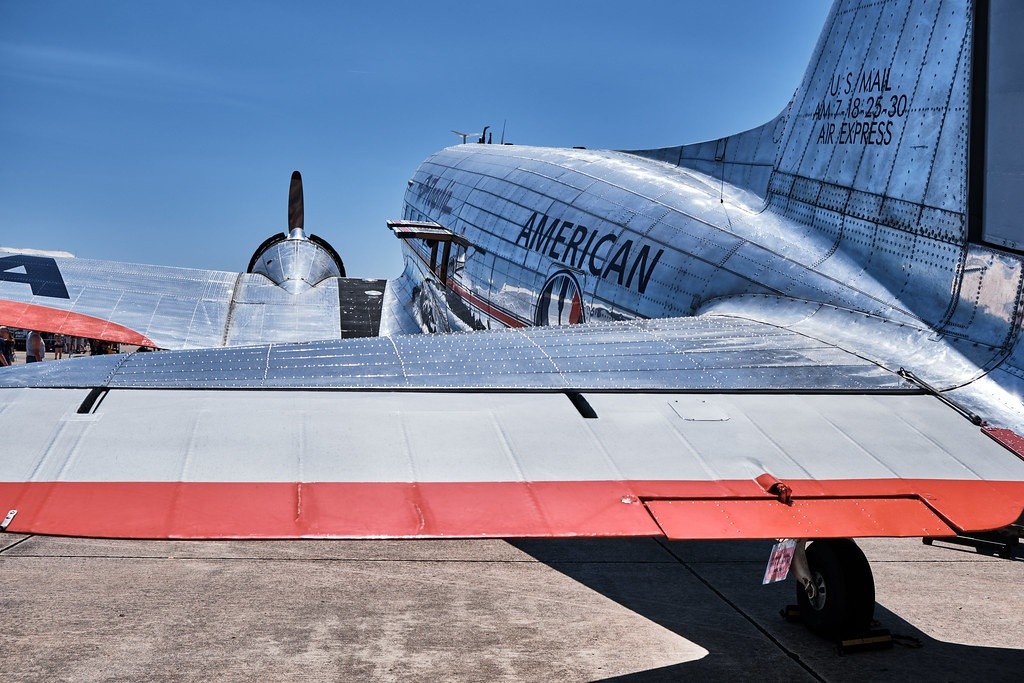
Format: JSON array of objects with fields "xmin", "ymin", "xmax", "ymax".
[{"xmin": 3, "ymin": 0, "xmax": 1023, "ymax": 645}]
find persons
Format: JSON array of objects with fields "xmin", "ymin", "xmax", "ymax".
[{"xmin": 0, "ymin": 325, "xmax": 114, "ymax": 367}]
